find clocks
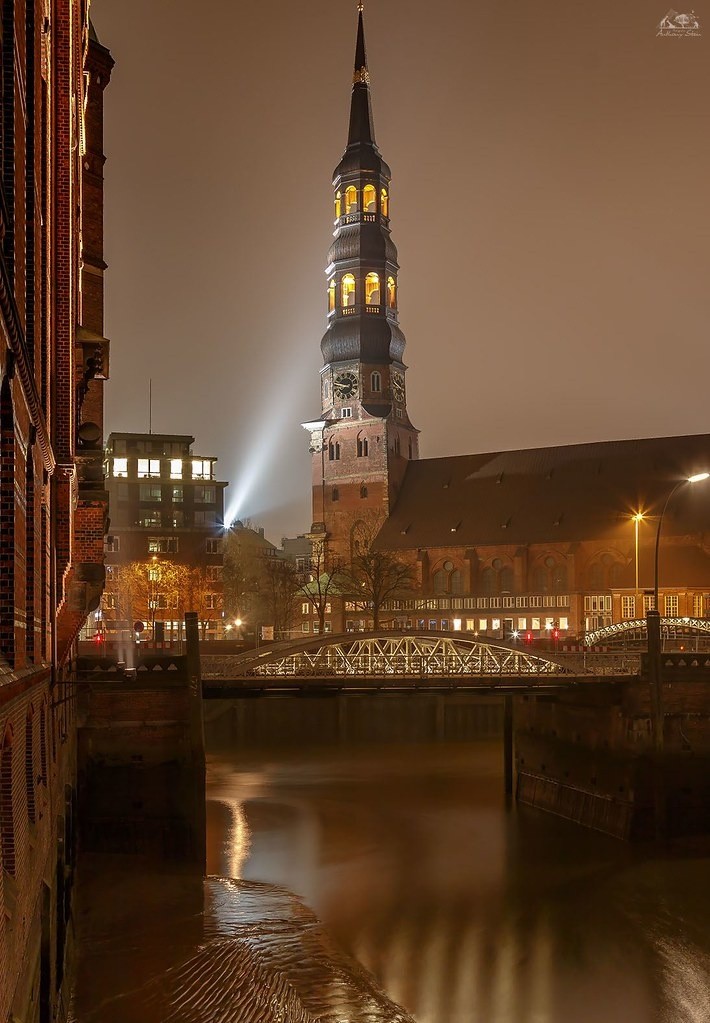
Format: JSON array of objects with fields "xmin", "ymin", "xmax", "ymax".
[
  {"xmin": 332, "ymin": 371, "xmax": 359, "ymax": 400},
  {"xmin": 392, "ymin": 374, "xmax": 405, "ymax": 402}
]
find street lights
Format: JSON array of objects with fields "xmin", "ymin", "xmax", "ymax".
[
  {"xmin": 632, "ymin": 512, "xmax": 645, "ymax": 617},
  {"xmin": 654, "ymin": 472, "xmax": 710, "ymax": 612},
  {"xmin": 233, "ymin": 618, "xmax": 242, "ymax": 640}
]
